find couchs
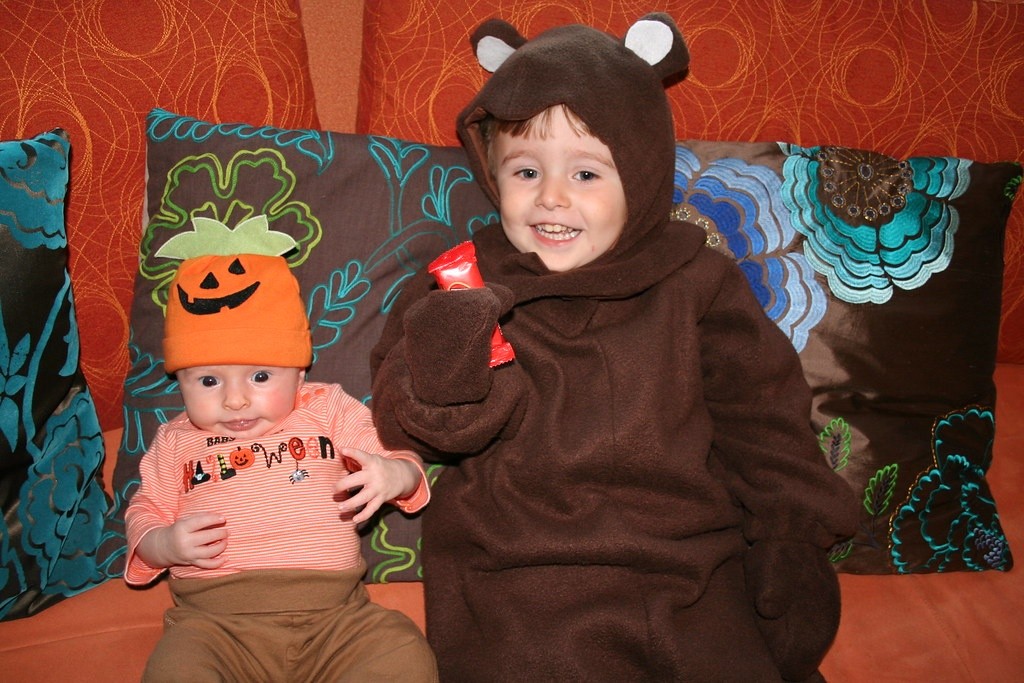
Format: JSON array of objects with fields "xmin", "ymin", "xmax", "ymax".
[{"xmin": 0, "ymin": 0, "xmax": 1024, "ymax": 683}]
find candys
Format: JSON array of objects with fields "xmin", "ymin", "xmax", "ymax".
[{"xmin": 428, "ymin": 240, "xmax": 517, "ymax": 369}]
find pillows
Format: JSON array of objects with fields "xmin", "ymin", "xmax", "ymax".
[
  {"xmin": 668, "ymin": 140, "xmax": 1024, "ymax": 574},
  {"xmin": 0, "ymin": 126, "xmax": 111, "ymax": 625},
  {"xmin": 95, "ymin": 105, "xmax": 499, "ymax": 584}
]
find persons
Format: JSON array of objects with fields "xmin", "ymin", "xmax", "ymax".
[
  {"xmin": 124, "ymin": 255, "xmax": 439, "ymax": 683},
  {"xmin": 370, "ymin": 13, "xmax": 858, "ymax": 683}
]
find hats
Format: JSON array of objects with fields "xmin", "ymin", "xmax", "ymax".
[{"xmin": 155, "ymin": 214, "xmax": 311, "ymax": 374}]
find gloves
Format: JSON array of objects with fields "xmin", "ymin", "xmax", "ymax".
[
  {"xmin": 750, "ymin": 539, "xmax": 841, "ymax": 681},
  {"xmin": 402, "ymin": 281, "xmax": 515, "ymax": 407}
]
